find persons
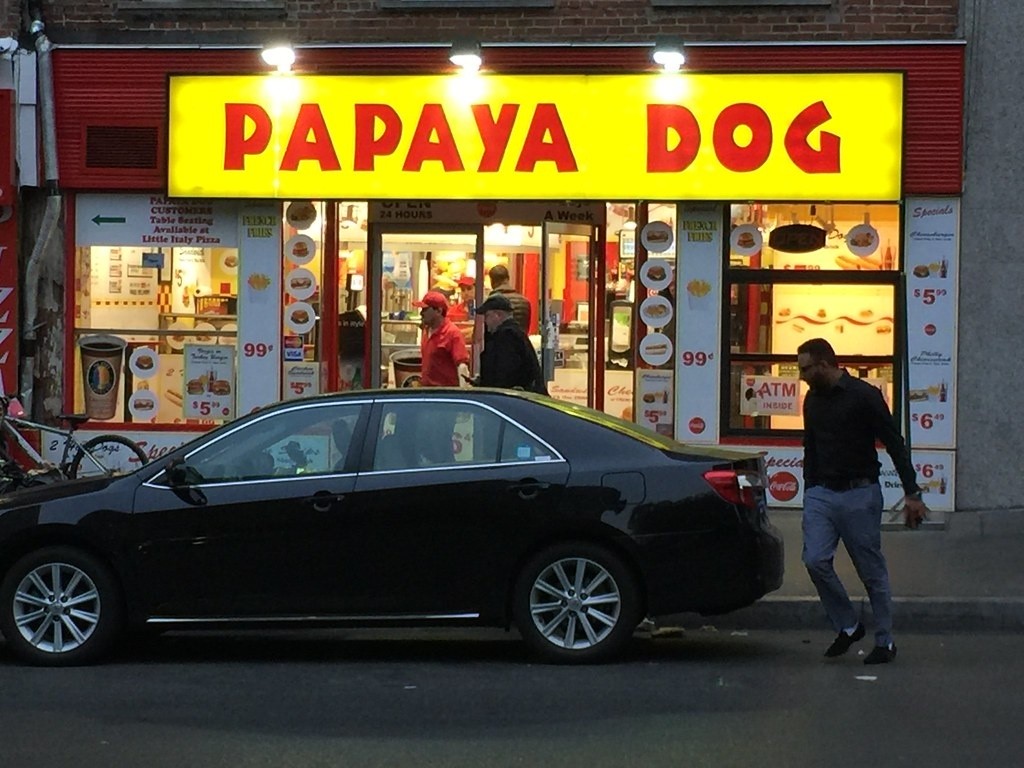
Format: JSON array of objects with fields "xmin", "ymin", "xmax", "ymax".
[
  {"xmin": 332, "ymin": 416, "xmax": 357, "ymax": 473},
  {"xmin": 444, "ymin": 276, "xmax": 478, "ymax": 364},
  {"xmin": 412, "ymin": 291, "xmax": 468, "ymax": 462},
  {"xmin": 490, "ymin": 264, "xmax": 532, "ymax": 335},
  {"xmin": 472, "ymin": 293, "xmax": 550, "ymax": 460},
  {"xmin": 798, "ymin": 337, "xmax": 929, "ymax": 667}
]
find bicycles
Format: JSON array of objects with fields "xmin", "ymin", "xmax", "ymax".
[{"xmin": 0, "ymin": 392, "xmax": 150, "ymax": 483}]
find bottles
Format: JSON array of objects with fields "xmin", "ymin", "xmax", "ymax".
[{"xmin": 884, "ymin": 247, "xmax": 892, "ymax": 269}]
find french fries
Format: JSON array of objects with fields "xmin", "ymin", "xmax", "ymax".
[
  {"xmin": 688, "ymin": 279, "xmax": 712, "ymax": 297},
  {"xmin": 248, "ymin": 273, "xmax": 268, "ymax": 290},
  {"xmin": 928, "ymin": 262, "xmax": 940, "ymax": 271}
]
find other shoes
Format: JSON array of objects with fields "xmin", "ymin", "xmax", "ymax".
[
  {"xmin": 824, "ymin": 622, "xmax": 866, "ymax": 657},
  {"xmin": 863, "ymin": 640, "xmax": 897, "ymax": 665}
]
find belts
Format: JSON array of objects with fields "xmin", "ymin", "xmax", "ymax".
[{"xmin": 816, "ymin": 477, "xmax": 871, "ymax": 491}]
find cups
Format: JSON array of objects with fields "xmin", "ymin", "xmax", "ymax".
[
  {"xmin": 938, "ymin": 477, "xmax": 948, "ymax": 494},
  {"xmin": 939, "ymin": 383, "xmax": 947, "ymax": 402},
  {"xmin": 390, "ymin": 349, "xmax": 424, "ymax": 389},
  {"xmin": 78, "ymin": 336, "xmax": 128, "ymax": 419},
  {"xmin": 206, "ymin": 370, "xmax": 217, "ymax": 392},
  {"xmin": 939, "ymin": 259, "xmax": 948, "ymax": 278}
]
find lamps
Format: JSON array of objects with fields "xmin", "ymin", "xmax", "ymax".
[
  {"xmin": 17, "ymin": 24, "xmax": 49, "ymax": 53},
  {"xmin": 447, "ymin": 35, "xmax": 485, "ymax": 69}
]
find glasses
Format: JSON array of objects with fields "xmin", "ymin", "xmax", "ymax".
[{"xmin": 797, "ymin": 360, "xmax": 829, "ymax": 373}]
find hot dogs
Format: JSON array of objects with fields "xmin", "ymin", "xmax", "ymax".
[
  {"xmin": 647, "ymin": 230, "xmax": 668, "ymax": 242},
  {"xmin": 133, "ymin": 399, "xmax": 155, "ymax": 410},
  {"xmin": 291, "ymin": 277, "xmax": 311, "ymax": 289},
  {"xmin": 645, "ymin": 343, "xmax": 666, "ymax": 354}
]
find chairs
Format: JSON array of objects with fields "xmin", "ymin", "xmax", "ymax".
[{"xmin": 329, "ymin": 435, "xmax": 408, "ymax": 473}]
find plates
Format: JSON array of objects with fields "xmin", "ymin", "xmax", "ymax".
[
  {"xmin": 218, "ymin": 324, "xmax": 238, "ymax": 351},
  {"xmin": 641, "ymin": 259, "xmax": 672, "ymax": 290},
  {"xmin": 286, "ymin": 268, "xmax": 317, "ymax": 300},
  {"xmin": 284, "ymin": 302, "xmax": 316, "ymax": 333},
  {"xmin": 846, "ymin": 225, "xmax": 879, "ymax": 257},
  {"xmin": 166, "ymin": 322, "xmax": 191, "ymax": 350},
  {"xmin": 639, "ymin": 333, "xmax": 673, "ymax": 366},
  {"xmin": 641, "ymin": 221, "xmax": 674, "ymax": 253},
  {"xmin": 129, "ymin": 390, "xmax": 158, "ymax": 420},
  {"xmin": 285, "ymin": 234, "xmax": 317, "ymax": 265},
  {"xmin": 730, "ymin": 224, "xmax": 763, "ymax": 257},
  {"xmin": 286, "ymin": 201, "xmax": 317, "ymax": 230},
  {"xmin": 129, "ymin": 348, "xmax": 160, "ymax": 378},
  {"xmin": 640, "ymin": 296, "xmax": 672, "ymax": 327},
  {"xmin": 193, "ymin": 323, "xmax": 217, "ymax": 345}
]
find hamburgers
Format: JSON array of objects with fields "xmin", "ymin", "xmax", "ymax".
[
  {"xmin": 187, "ymin": 379, "xmax": 204, "ymax": 395},
  {"xmin": 213, "ymin": 380, "xmax": 230, "ymax": 395},
  {"xmin": 913, "ymin": 265, "xmax": 930, "ymax": 277},
  {"xmin": 291, "ymin": 310, "xmax": 309, "ymax": 323},
  {"xmin": 647, "ymin": 266, "xmax": 666, "ymax": 281},
  {"xmin": 643, "ymin": 393, "xmax": 655, "ymax": 403},
  {"xmin": 737, "ymin": 232, "xmax": 755, "ymax": 248},
  {"xmin": 292, "ymin": 242, "xmax": 308, "ymax": 257},
  {"xmin": 135, "ymin": 356, "xmax": 154, "ymax": 369}
]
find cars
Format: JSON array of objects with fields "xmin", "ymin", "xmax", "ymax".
[{"xmin": 0, "ymin": 383, "xmax": 785, "ymax": 665}]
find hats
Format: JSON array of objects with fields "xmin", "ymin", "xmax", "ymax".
[
  {"xmin": 475, "ymin": 296, "xmax": 515, "ymax": 313},
  {"xmin": 413, "ymin": 291, "xmax": 449, "ymax": 318}
]
